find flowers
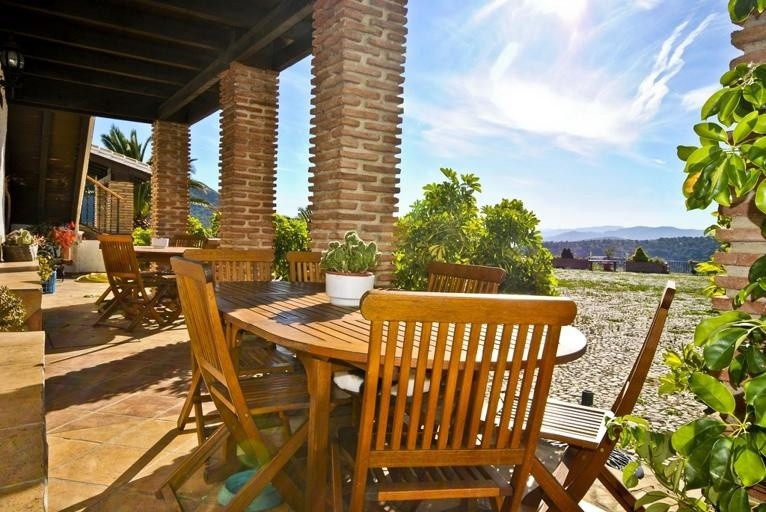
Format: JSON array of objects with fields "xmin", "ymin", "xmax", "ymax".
[{"xmin": 51, "ymin": 220, "xmax": 79, "ymax": 248}]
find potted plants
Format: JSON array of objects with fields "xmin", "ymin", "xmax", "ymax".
[
  {"xmin": 316, "ymin": 227, "xmax": 384, "ymax": 308},
  {"xmin": 0, "ymin": 227, "xmax": 56, "ymax": 295}
]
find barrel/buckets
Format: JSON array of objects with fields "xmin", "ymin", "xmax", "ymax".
[{"xmin": 73, "ymin": 240, "xmax": 100, "ymax": 272}]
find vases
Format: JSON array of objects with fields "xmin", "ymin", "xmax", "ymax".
[{"xmin": 60, "ymin": 248, "xmax": 72, "ymax": 263}]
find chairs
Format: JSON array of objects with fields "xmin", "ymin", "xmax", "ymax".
[{"xmin": 89, "ymin": 229, "xmax": 222, "ymax": 333}]
